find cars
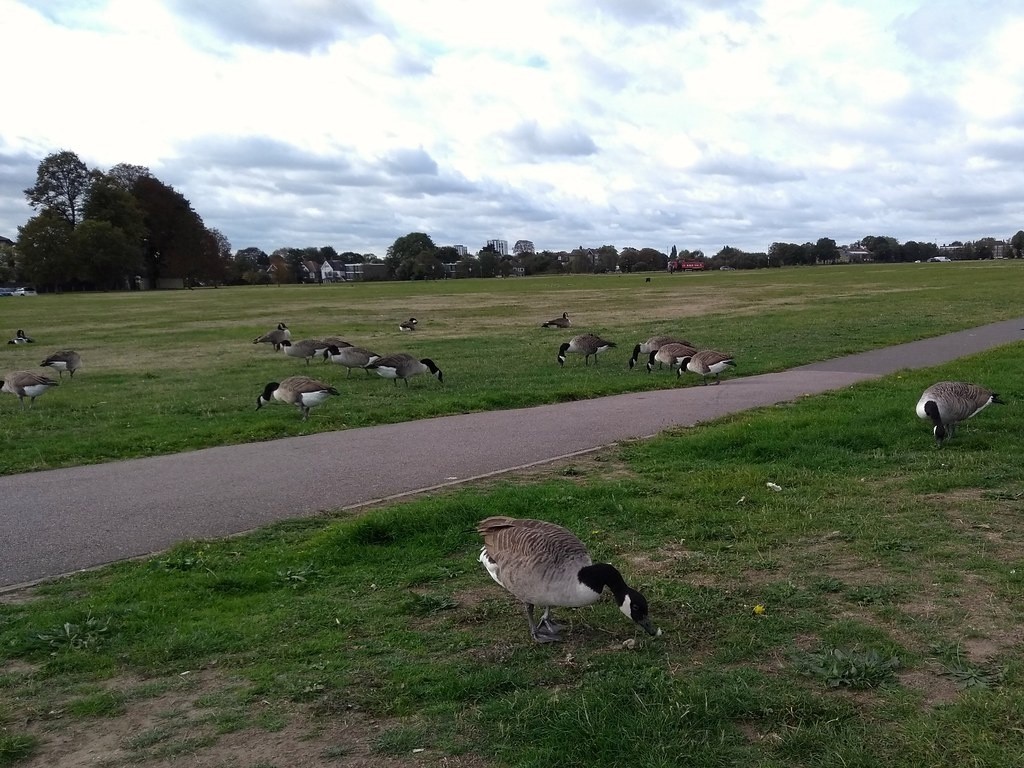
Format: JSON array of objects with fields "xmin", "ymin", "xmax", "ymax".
[
  {"xmin": 720, "ymin": 266, "xmax": 736, "ymax": 271},
  {"xmin": 927, "ymin": 258, "xmax": 941, "ymax": 262},
  {"xmin": 0, "ymin": 287, "xmax": 38, "ymax": 297}
]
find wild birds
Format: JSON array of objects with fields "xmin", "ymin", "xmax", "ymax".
[
  {"xmin": 256, "ymin": 376, "xmax": 338, "ymax": 424},
  {"xmin": 398, "ymin": 318, "xmax": 419, "ymax": 327},
  {"xmin": 0, "ymin": 370, "xmax": 60, "ymax": 409},
  {"xmin": 543, "ymin": 312, "xmax": 572, "ymax": 327},
  {"xmin": 39, "ymin": 350, "xmax": 84, "ymax": 380},
  {"xmin": 676, "ymin": 350, "xmax": 737, "ymax": 381},
  {"xmin": 628, "ymin": 334, "xmax": 693, "ymax": 372},
  {"xmin": 916, "ymin": 380, "xmax": 1005, "ymax": 442},
  {"xmin": 557, "ymin": 332, "xmax": 618, "ymax": 368},
  {"xmin": 646, "ymin": 343, "xmax": 700, "ymax": 374},
  {"xmin": 251, "ymin": 322, "xmax": 291, "ymax": 350},
  {"xmin": 472, "ymin": 515, "xmax": 661, "ymax": 643},
  {"xmin": 7, "ymin": 330, "xmax": 37, "ymax": 348},
  {"xmin": 277, "ymin": 336, "xmax": 444, "ymax": 389}
]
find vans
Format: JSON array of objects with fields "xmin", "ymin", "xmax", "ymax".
[{"xmin": 934, "ymin": 257, "xmax": 951, "ymax": 262}]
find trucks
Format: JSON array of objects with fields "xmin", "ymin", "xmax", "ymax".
[{"xmin": 667, "ymin": 260, "xmax": 705, "ymax": 272}]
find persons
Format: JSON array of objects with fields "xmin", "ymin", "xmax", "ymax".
[
  {"xmin": 669, "ymin": 263, "xmax": 674, "ymax": 274},
  {"xmin": 616, "ymin": 265, "xmax": 620, "ymax": 274},
  {"xmin": 9, "ymin": 330, "xmax": 33, "ymax": 343}
]
